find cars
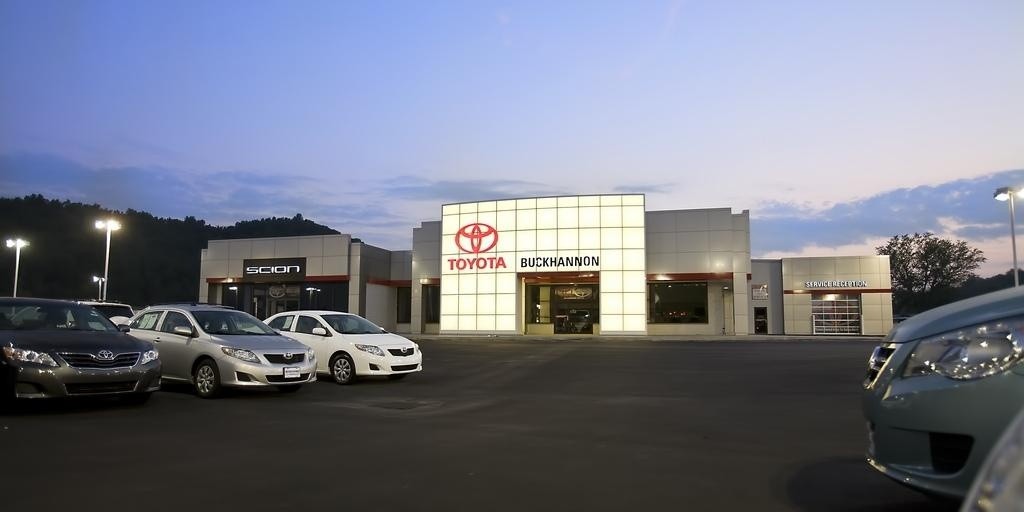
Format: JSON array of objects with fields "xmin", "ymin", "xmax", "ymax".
[
  {"xmin": 665, "ymin": 305, "xmax": 689, "ymax": 322},
  {"xmin": 555, "ymin": 310, "xmax": 591, "ymax": 334},
  {"xmin": 860, "ymin": 286, "xmax": 1024, "ymax": 512}
]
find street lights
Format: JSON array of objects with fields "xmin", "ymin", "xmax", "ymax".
[
  {"xmin": 95, "ymin": 220, "xmax": 119, "ymax": 302},
  {"xmin": 7, "ymin": 239, "xmax": 30, "ymax": 297},
  {"xmin": 994, "ymin": 186, "xmax": 1024, "ymax": 286},
  {"xmin": 93, "ymin": 276, "xmax": 105, "ymax": 300}
]
